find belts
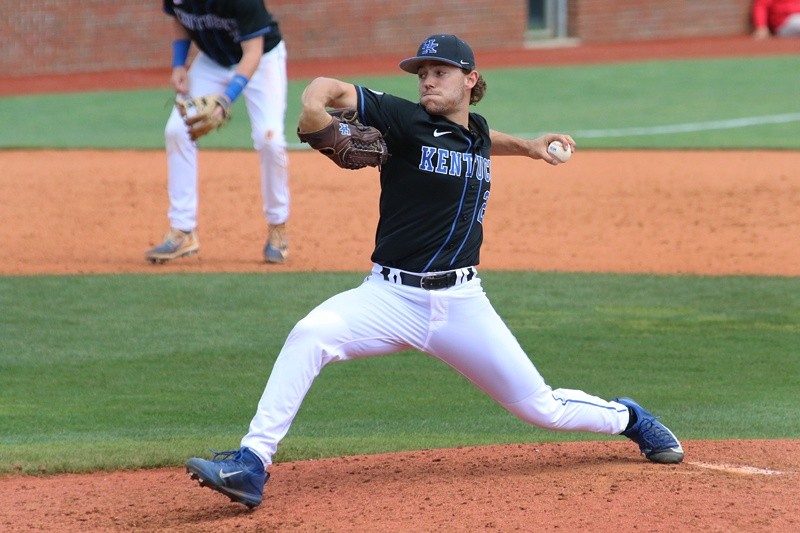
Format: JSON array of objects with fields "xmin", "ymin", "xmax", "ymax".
[{"xmin": 377, "ymin": 265, "xmax": 474, "ymax": 289}]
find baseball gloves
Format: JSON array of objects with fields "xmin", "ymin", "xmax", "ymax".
[
  {"xmin": 294, "ymin": 105, "xmax": 394, "ymax": 173},
  {"xmin": 175, "ymin": 93, "xmax": 232, "ymax": 141}
]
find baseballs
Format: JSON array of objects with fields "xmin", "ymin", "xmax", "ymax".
[{"xmin": 547, "ymin": 140, "xmax": 571, "ymax": 163}]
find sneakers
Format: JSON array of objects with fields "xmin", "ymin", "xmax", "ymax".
[
  {"xmin": 263, "ymin": 224, "xmax": 288, "ymax": 262},
  {"xmin": 148, "ymin": 228, "xmax": 200, "ymax": 264},
  {"xmin": 612, "ymin": 395, "xmax": 686, "ymax": 463},
  {"xmin": 186, "ymin": 445, "xmax": 270, "ymax": 508}
]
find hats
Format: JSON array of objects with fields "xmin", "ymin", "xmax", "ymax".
[{"xmin": 400, "ymin": 33, "xmax": 476, "ymax": 75}]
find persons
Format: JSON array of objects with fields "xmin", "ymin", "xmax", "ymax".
[
  {"xmin": 750, "ymin": 0, "xmax": 800, "ymax": 39},
  {"xmin": 144, "ymin": 0, "xmax": 290, "ymax": 264},
  {"xmin": 185, "ymin": 37, "xmax": 684, "ymax": 511}
]
